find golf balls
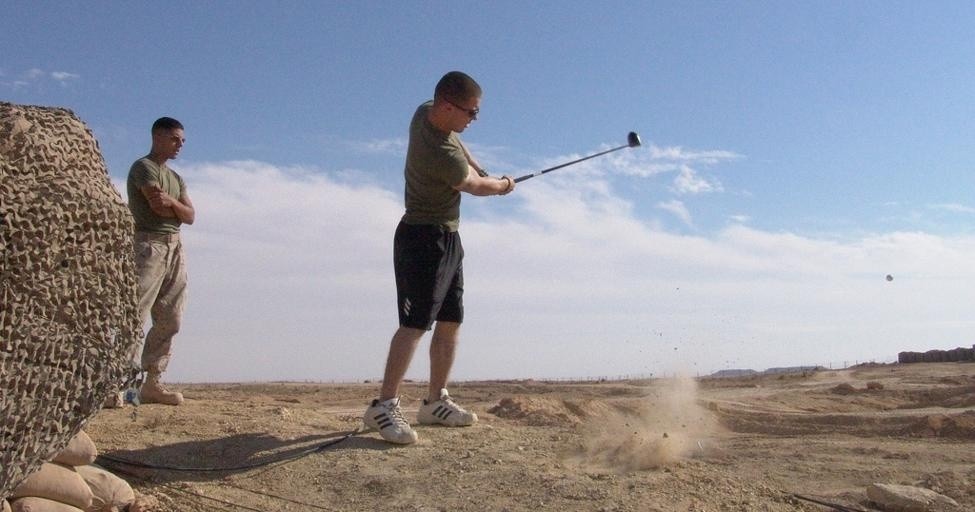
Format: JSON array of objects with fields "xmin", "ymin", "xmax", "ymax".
[{"xmin": 886, "ymin": 274, "xmax": 895, "ymax": 283}]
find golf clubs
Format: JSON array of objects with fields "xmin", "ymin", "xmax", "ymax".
[{"xmin": 514, "ymin": 132, "xmax": 641, "ymax": 182}]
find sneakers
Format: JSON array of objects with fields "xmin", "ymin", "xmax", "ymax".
[
  {"xmin": 103, "ymin": 392, "xmax": 125, "ymax": 408},
  {"xmin": 140, "ymin": 379, "xmax": 184, "ymax": 406},
  {"xmin": 363, "ymin": 395, "xmax": 418, "ymax": 445},
  {"xmin": 416, "ymin": 387, "xmax": 479, "ymax": 427}
]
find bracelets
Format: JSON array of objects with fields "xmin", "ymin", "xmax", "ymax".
[{"xmin": 501, "ymin": 176, "xmax": 511, "ymax": 193}]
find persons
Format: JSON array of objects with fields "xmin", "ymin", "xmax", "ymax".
[
  {"xmin": 126, "ymin": 116, "xmax": 195, "ymax": 406},
  {"xmin": 361, "ymin": 70, "xmax": 515, "ymax": 446}
]
